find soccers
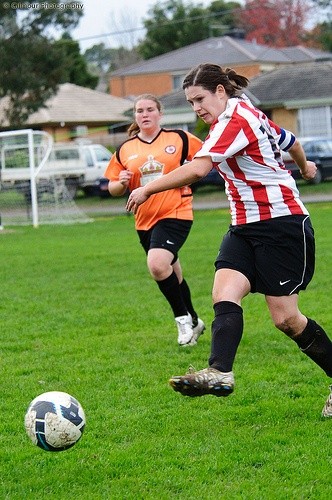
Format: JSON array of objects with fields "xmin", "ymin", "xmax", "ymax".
[{"xmin": 25, "ymin": 391, "xmax": 85, "ymax": 452}]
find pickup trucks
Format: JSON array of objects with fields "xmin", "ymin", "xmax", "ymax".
[{"xmin": 3, "ymin": 143, "xmax": 118, "ymax": 200}]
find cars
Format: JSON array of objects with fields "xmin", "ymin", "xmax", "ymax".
[
  {"xmin": 282, "ymin": 135, "xmax": 332, "ymax": 183},
  {"xmin": 95, "ymin": 164, "xmax": 113, "ymax": 198}
]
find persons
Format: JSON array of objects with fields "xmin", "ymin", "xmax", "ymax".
[
  {"xmin": 104, "ymin": 94, "xmax": 204, "ymax": 347},
  {"xmin": 123, "ymin": 63, "xmax": 332, "ymax": 418}
]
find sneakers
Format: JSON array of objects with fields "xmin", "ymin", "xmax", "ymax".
[
  {"xmin": 169, "ymin": 368, "xmax": 235, "ymax": 399},
  {"xmin": 173, "ymin": 314, "xmax": 208, "ymax": 349},
  {"xmin": 322, "ymin": 378, "xmax": 332, "ymax": 419}
]
